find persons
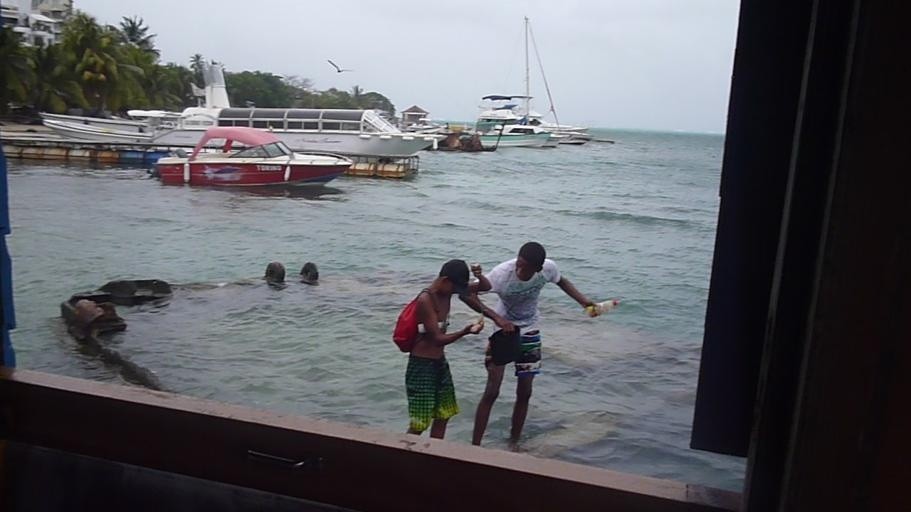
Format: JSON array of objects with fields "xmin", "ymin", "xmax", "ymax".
[
  {"xmin": 458, "ymin": 241, "xmax": 601, "ymax": 453},
  {"xmin": 404, "ymin": 259, "xmax": 491, "ymax": 440}
]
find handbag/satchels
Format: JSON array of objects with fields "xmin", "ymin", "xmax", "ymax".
[{"xmin": 391, "ymin": 287, "xmax": 438, "ymax": 352}]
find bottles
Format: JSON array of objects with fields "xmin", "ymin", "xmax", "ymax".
[
  {"xmin": 584, "ymin": 299, "xmax": 619, "ymax": 319},
  {"xmin": 416, "ymin": 321, "xmax": 447, "ymax": 333}
]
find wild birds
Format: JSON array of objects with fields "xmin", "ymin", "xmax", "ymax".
[{"xmin": 328, "ymin": 59, "xmax": 353, "ymax": 72}]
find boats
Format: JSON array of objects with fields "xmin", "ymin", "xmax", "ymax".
[
  {"xmin": 468, "ymin": 13, "xmax": 592, "ymax": 148},
  {"xmin": 151, "ymin": 125, "xmax": 356, "ymax": 192}
]
